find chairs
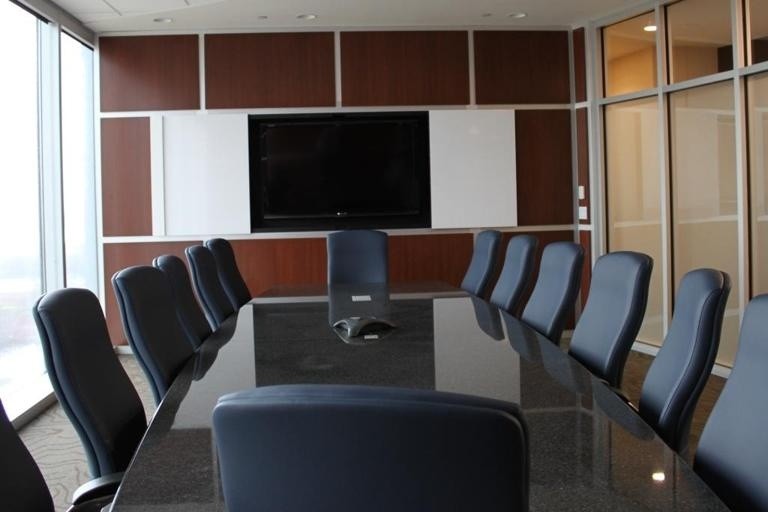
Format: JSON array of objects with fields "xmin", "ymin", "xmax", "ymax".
[
  {"xmin": 184, "ymin": 245, "xmax": 234, "ymax": 331},
  {"xmin": 153, "ymin": 255, "xmax": 212, "ymax": 351},
  {"xmin": 461, "ymin": 230, "xmax": 502, "ymax": 297},
  {"xmin": 489, "ymin": 233, "xmax": 538, "ymax": 316},
  {"xmin": 610, "ymin": 266, "xmax": 732, "ymax": 461},
  {"xmin": 205, "ymin": 238, "xmax": 253, "ymax": 312},
  {"xmin": 692, "ymin": 293, "xmax": 768, "ymax": 512},
  {"xmin": 111, "ymin": 264, "xmax": 193, "ymax": 406},
  {"xmin": 326, "ymin": 230, "xmax": 389, "ymax": 284},
  {"xmin": 570, "ymin": 251, "xmax": 653, "ymax": 389},
  {"xmin": 213, "ymin": 382, "xmax": 531, "ymax": 511},
  {"xmin": 519, "ymin": 241, "xmax": 585, "ymax": 346},
  {"xmin": 0, "ymin": 399, "xmax": 124, "ymax": 512},
  {"xmin": 32, "ymin": 288, "xmax": 147, "ymax": 478}
]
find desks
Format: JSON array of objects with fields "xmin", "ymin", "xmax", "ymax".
[{"xmin": 109, "ymin": 280, "xmax": 730, "ymax": 512}]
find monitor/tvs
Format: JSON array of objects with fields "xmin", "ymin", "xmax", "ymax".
[{"xmin": 247, "ymin": 111, "xmax": 432, "ymax": 234}]
